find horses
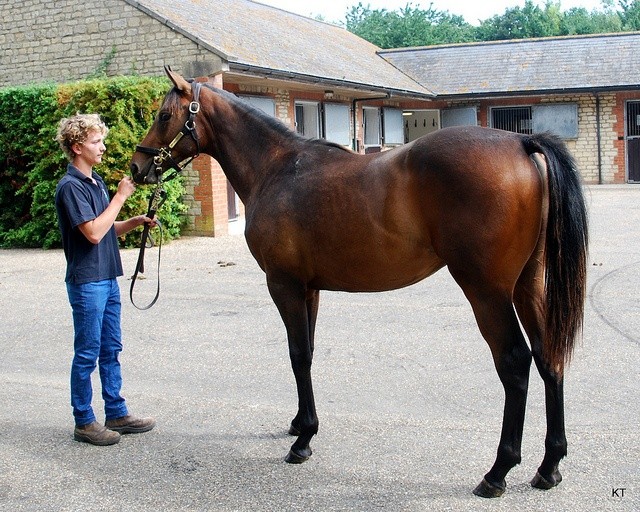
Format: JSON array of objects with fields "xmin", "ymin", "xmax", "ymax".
[{"xmin": 129, "ymin": 64, "xmax": 589, "ymax": 498}]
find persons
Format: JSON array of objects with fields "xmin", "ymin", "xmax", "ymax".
[{"xmin": 55, "ymin": 113, "xmax": 158, "ymax": 446}]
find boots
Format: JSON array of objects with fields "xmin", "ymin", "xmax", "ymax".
[
  {"xmin": 74, "ymin": 409, "xmax": 120, "ymax": 446},
  {"xmin": 104, "ymin": 406, "xmax": 156, "ymax": 434}
]
